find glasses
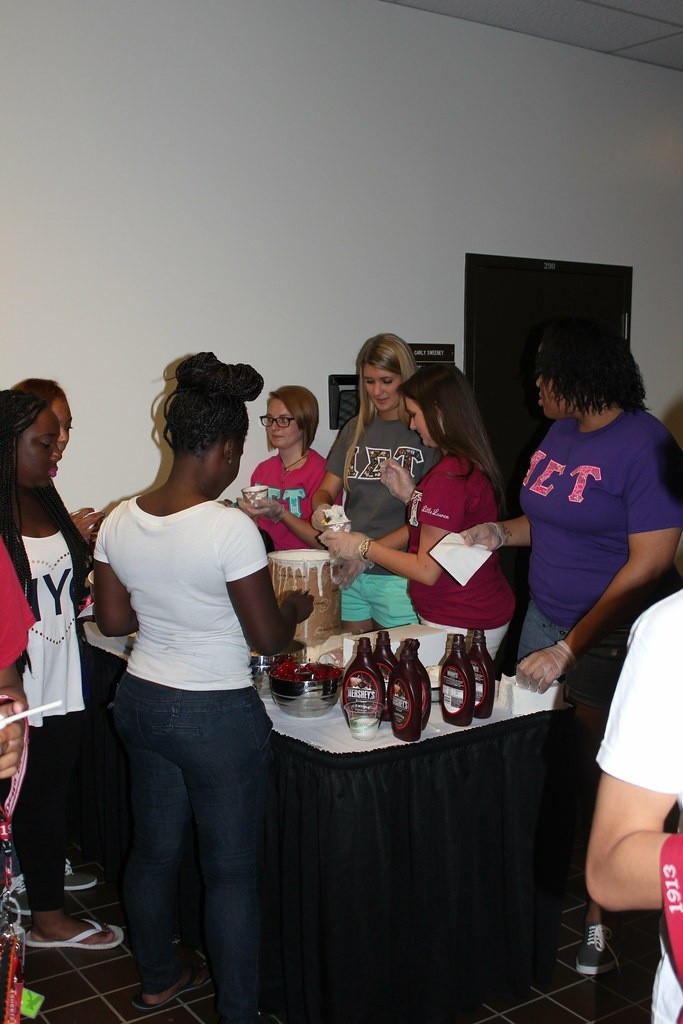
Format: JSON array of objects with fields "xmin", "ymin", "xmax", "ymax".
[{"xmin": 259, "ymin": 415, "xmax": 295, "ymax": 428}]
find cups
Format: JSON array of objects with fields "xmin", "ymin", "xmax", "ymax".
[
  {"xmin": 241, "ymin": 485, "xmax": 269, "ymax": 509},
  {"xmin": 319, "ymin": 647, "xmax": 342, "ymax": 667},
  {"xmin": 87, "ymin": 570, "xmax": 94, "ymax": 602},
  {"xmin": 323, "ymin": 522, "xmax": 352, "ymax": 533}
]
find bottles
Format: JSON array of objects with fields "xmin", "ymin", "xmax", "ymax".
[
  {"xmin": 388, "ymin": 647, "xmax": 421, "ymax": 742},
  {"xmin": 404, "ymin": 638, "xmax": 432, "ymax": 731},
  {"xmin": 440, "ymin": 634, "xmax": 475, "ymax": 726},
  {"xmin": 344, "ymin": 701, "xmax": 384, "ymax": 728},
  {"xmin": 343, "ymin": 637, "xmax": 386, "ymax": 728},
  {"xmin": 374, "ymin": 631, "xmax": 398, "ymax": 722}
]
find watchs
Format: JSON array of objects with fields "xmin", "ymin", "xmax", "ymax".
[{"xmin": 361, "ymin": 537, "xmax": 374, "ymax": 560}]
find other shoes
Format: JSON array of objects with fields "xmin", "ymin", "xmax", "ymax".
[{"xmin": 64, "ymin": 858, "xmax": 98, "ymax": 890}]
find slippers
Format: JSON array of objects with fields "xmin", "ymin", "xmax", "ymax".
[
  {"xmin": 131, "ymin": 950, "xmax": 212, "ymax": 1011},
  {"xmin": 24, "ymin": 918, "xmax": 124, "ymax": 950}
]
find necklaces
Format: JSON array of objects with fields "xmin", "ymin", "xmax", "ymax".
[{"xmin": 281, "ymin": 452, "xmax": 308, "ymax": 472}]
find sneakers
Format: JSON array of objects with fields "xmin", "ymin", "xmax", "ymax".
[
  {"xmin": 576, "ymin": 919, "xmax": 622, "ymax": 975},
  {"xmin": 3, "ymin": 874, "xmax": 31, "ymax": 915}
]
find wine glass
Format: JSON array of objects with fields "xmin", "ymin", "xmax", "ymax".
[{"xmin": 467, "ymin": 629, "xmax": 495, "ymax": 719}]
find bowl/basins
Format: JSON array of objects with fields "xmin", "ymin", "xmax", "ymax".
[
  {"xmin": 425, "ymin": 665, "xmax": 443, "ymax": 702},
  {"xmin": 268, "ymin": 663, "xmax": 344, "ymax": 718}
]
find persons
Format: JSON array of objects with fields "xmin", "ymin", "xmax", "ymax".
[
  {"xmin": 458, "ymin": 320, "xmax": 683, "ymax": 974},
  {"xmin": 220, "ymin": 332, "xmax": 514, "ymax": 664},
  {"xmin": 587, "ymin": 585, "xmax": 683, "ymax": 1024},
  {"xmin": 91, "ymin": 348, "xmax": 313, "ymax": 1024},
  {"xmin": 0, "ymin": 377, "xmax": 124, "ymax": 1024}
]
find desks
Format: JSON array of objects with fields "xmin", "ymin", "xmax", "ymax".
[{"xmin": 64, "ymin": 620, "xmax": 577, "ymax": 1024}]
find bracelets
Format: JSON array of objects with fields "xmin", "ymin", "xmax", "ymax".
[
  {"xmin": 359, "ymin": 538, "xmax": 372, "ymax": 561},
  {"xmin": 488, "ymin": 522, "xmax": 504, "ymax": 549}
]
